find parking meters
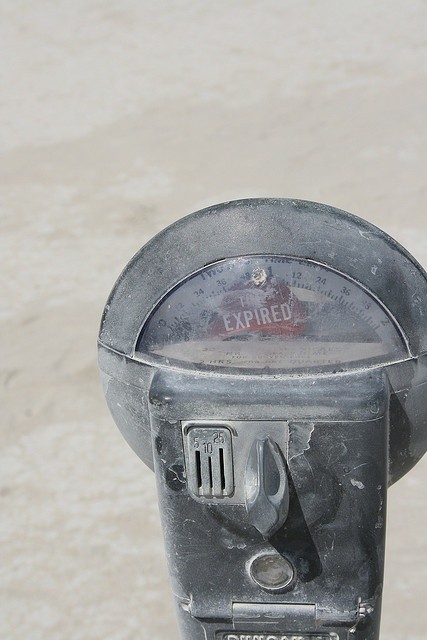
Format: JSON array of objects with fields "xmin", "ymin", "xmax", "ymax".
[{"xmin": 96, "ymin": 199, "xmax": 427, "ymax": 640}]
